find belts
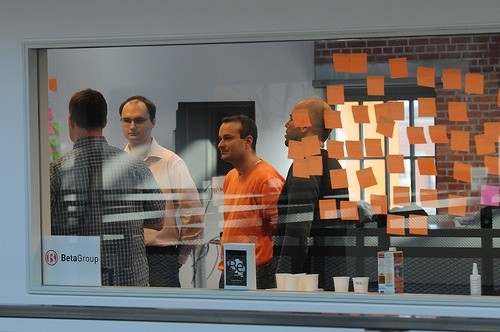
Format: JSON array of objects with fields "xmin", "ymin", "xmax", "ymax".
[{"xmin": 256, "ymin": 260, "xmax": 271, "ymax": 273}]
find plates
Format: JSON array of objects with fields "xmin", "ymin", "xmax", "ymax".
[{"xmin": 265, "ymin": 288, "xmax": 324, "ymax": 291}]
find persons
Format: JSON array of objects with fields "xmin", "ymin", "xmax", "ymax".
[
  {"xmin": 51, "ymin": 88, "xmax": 166, "ymax": 286},
  {"xmin": 118, "ymin": 96, "xmax": 205, "ymax": 287},
  {"xmin": 218, "ymin": 114, "xmax": 286, "ymax": 289},
  {"xmin": 271, "ymin": 98, "xmax": 350, "ymax": 291}
]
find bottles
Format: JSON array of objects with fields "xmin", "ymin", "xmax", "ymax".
[{"xmin": 470, "ymin": 263, "xmax": 481, "ymax": 296}]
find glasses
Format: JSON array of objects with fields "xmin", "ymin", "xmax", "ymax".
[{"xmin": 120, "ymin": 118, "xmax": 150, "ymax": 124}]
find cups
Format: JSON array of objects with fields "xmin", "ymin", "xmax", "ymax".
[
  {"xmin": 333, "ymin": 277, "xmax": 350, "ymax": 292},
  {"xmin": 276, "ymin": 273, "xmax": 318, "ymax": 290},
  {"xmin": 352, "ymin": 277, "xmax": 369, "ymax": 293}
]
